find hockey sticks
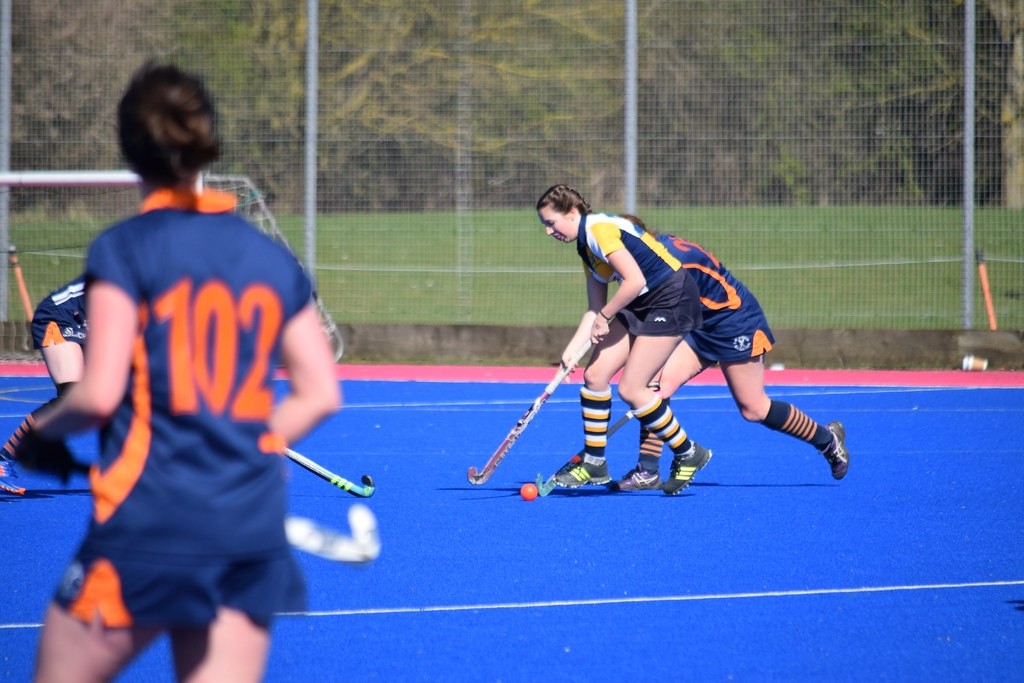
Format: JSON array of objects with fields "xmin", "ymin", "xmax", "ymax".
[
  {"xmin": 284, "ymin": 445, "xmax": 377, "ymax": 500},
  {"xmin": 536, "ymin": 410, "xmax": 635, "ymax": 498},
  {"xmin": 467, "ymin": 314, "xmax": 617, "ymax": 484},
  {"xmin": 18, "ymin": 438, "xmax": 382, "ymax": 564}
]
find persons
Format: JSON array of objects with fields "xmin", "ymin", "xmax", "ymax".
[
  {"xmin": 612, "ymin": 214, "xmax": 849, "ymax": 491},
  {"xmin": 11, "ymin": 62, "xmax": 344, "ymax": 683},
  {"xmin": 536, "ymin": 186, "xmax": 712, "ymax": 495},
  {"xmin": 1, "ymin": 275, "xmax": 87, "ymax": 497}
]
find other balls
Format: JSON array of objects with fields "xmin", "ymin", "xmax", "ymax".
[{"xmin": 520, "ymin": 482, "xmax": 539, "ymax": 502}]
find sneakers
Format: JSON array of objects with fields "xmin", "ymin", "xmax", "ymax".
[
  {"xmin": 552, "ymin": 460, "xmax": 612, "ymax": 488},
  {"xmin": 0, "ymin": 461, "xmax": 25, "ymax": 495},
  {"xmin": 663, "ymin": 443, "xmax": 713, "ymax": 495},
  {"xmin": 819, "ymin": 421, "xmax": 849, "ymax": 480},
  {"xmin": 609, "ymin": 463, "xmax": 664, "ymax": 491}
]
